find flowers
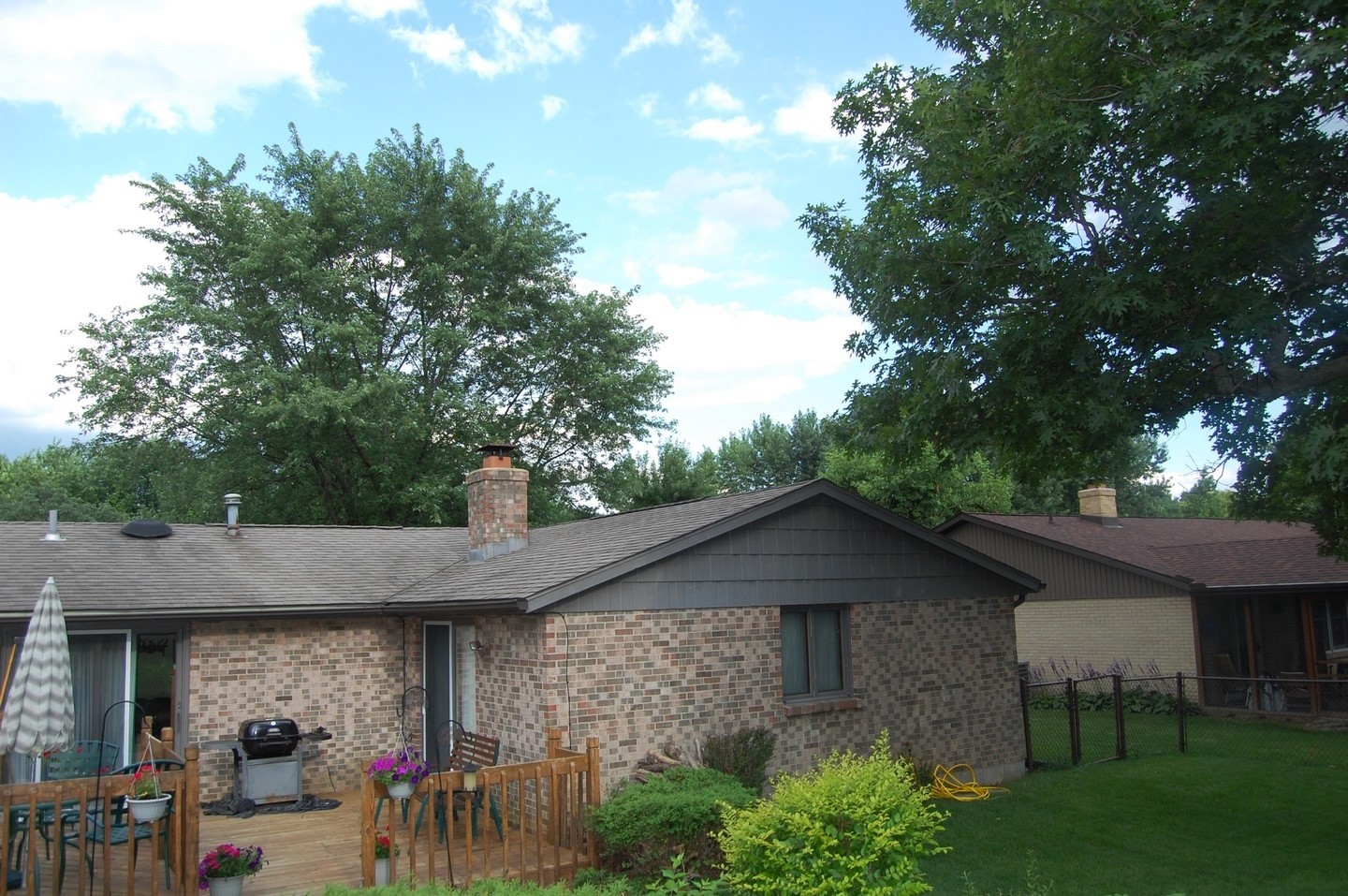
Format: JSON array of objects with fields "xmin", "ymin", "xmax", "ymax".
[
  {"xmin": 199, "ymin": 844, "xmax": 269, "ymax": 889},
  {"xmin": 128, "ymin": 764, "xmax": 163, "ymax": 801},
  {"xmin": 366, "ymin": 746, "xmax": 435, "ymax": 788},
  {"xmin": 372, "ymin": 825, "xmax": 401, "ymax": 859}
]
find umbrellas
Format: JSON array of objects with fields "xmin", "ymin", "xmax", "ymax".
[{"xmin": 0, "ymin": 577, "xmax": 74, "ymax": 783}]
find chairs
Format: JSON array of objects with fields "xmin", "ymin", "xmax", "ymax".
[
  {"xmin": 401, "ymin": 730, "xmax": 503, "ymax": 854},
  {"xmin": 17, "ymin": 740, "xmax": 186, "ymax": 896}
]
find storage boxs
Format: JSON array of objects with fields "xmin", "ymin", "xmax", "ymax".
[{"xmin": 236, "ymin": 718, "xmax": 300, "ymax": 755}]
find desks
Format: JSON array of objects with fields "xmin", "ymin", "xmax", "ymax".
[{"xmin": 0, "ymin": 797, "xmax": 94, "ymax": 896}]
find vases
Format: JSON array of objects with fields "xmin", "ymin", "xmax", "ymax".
[
  {"xmin": 205, "ymin": 873, "xmax": 245, "ymax": 896},
  {"xmin": 375, "ymin": 856, "xmax": 398, "ymax": 887},
  {"xmin": 125, "ymin": 793, "xmax": 172, "ymax": 823},
  {"xmin": 388, "ymin": 781, "xmax": 414, "ymax": 799}
]
